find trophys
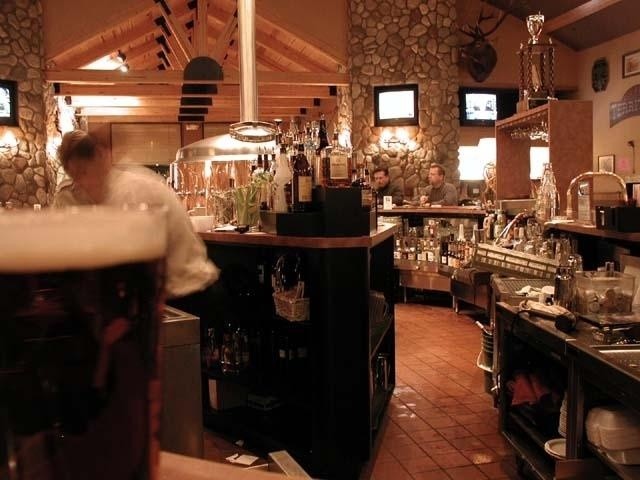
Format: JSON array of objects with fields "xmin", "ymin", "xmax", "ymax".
[{"xmin": 524, "ymin": 14, "xmax": 549, "ymax": 44}]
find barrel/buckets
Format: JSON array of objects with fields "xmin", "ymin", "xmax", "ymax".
[{"xmin": 476, "ymin": 333, "xmax": 494, "ymax": 394}]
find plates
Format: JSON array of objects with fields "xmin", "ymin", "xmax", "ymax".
[
  {"xmin": 558, "ymin": 397, "xmax": 567, "ymax": 438},
  {"xmin": 586, "ymin": 408, "xmax": 640, "ymax": 451},
  {"xmin": 544, "ymin": 438, "xmax": 568, "ymax": 460}
]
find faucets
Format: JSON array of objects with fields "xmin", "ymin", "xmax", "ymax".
[{"xmin": 566, "ymin": 172, "xmax": 628, "ymax": 221}]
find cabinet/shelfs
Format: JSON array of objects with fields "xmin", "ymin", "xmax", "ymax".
[
  {"xmin": 195, "ymin": 215, "xmax": 400, "ymax": 478},
  {"xmin": 375, "ymin": 99, "xmax": 640, "ymax": 480}
]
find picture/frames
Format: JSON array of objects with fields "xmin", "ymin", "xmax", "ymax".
[{"xmin": 622, "ymin": 49, "xmax": 640, "ymax": 79}]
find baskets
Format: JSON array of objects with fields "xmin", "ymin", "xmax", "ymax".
[{"xmin": 272, "ymin": 271, "xmax": 310, "ymax": 322}]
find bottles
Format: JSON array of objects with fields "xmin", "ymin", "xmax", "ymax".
[
  {"xmin": 483, "ymin": 209, "xmax": 572, "ymax": 260},
  {"xmin": 204, "ymin": 323, "xmax": 252, "ymax": 412},
  {"xmin": 374, "ymin": 352, "xmax": 388, "ymax": 391},
  {"xmin": 393, "ymin": 218, "xmax": 487, "ymax": 268},
  {"xmin": 257, "ymin": 111, "xmax": 372, "ymax": 212},
  {"xmin": 556, "ymin": 253, "xmax": 583, "ymax": 310},
  {"xmin": 578, "ymin": 182, "xmax": 591, "ymax": 225},
  {"xmin": 540, "ymin": 162, "xmax": 557, "ymax": 219}
]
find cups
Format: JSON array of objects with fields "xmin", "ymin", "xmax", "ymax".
[
  {"xmin": 370, "ymin": 291, "xmax": 389, "ymax": 325},
  {"xmin": 539, "ymin": 285, "xmax": 555, "ymax": 306},
  {"xmin": 187, "ymin": 207, "xmax": 207, "ymax": 217},
  {"xmin": 2, "ymin": 205, "xmax": 169, "ymax": 478}
]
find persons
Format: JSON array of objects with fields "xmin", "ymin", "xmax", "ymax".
[
  {"xmin": 47, "ymin": 130, "xmax": 221, "ymax": 377},
  {"xmin": 412, "ymin": 164, "xmax": 457, "ymax": 205},
  {"xmin": 373, "ymin": 167, "xmax": 403, "ymax": 206}
]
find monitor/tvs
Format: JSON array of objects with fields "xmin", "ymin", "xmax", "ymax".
[
  {"xmin": 374, "ymin": 83, "xmax": 418, "ymax": 127},
  {"xmin": 0, "ymin": 79, "xmax": 19, "ymax": 126},
  {"xmin": 459, "ymin": 86, "xmax": 503, "ymax": 126}
]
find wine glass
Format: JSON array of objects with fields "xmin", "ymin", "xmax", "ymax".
[
  {"xmin": 526, "ymin": 15, "xmax": 546, "ymax": 45},
  {"xmin": 510, "ymin": 118, "xmax": 550, "ymax": 144}
]
